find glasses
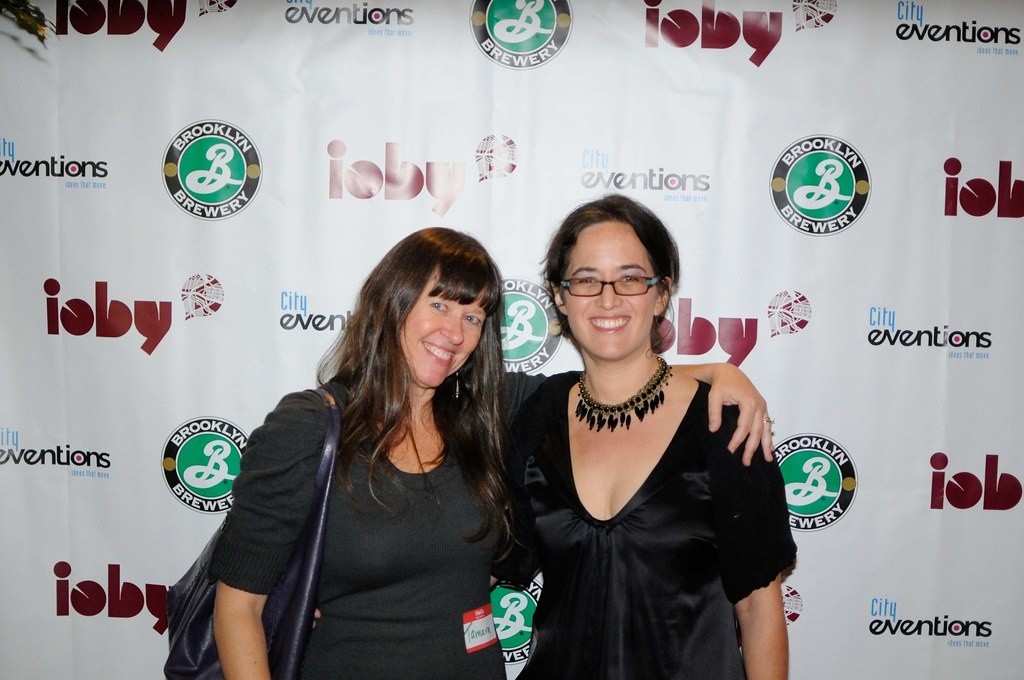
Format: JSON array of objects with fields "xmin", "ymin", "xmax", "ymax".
[{"xmin": 559, "ymin": 275, "xmax": 662, "ymax": 297}]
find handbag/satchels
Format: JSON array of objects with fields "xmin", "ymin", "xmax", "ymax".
[{"xmin": 164, "ymin": 388, "xmax": 340, "ymax": 680}]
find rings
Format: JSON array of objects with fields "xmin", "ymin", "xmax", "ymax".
[{"xmin": 762, "ymin": 417, "xmax": 776, "ymax": 424}]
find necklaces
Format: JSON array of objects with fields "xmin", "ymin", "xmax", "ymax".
[{"xmin": 574, "ymin": 356, "xmax": 673, "ymax": 433}]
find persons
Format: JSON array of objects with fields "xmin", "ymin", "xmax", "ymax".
[
  {"xmin": 311, "ymin": 195, "xmax": 798, "ymax": 680},
  {"xmin": 208, "ymin": 228, "xmax": 774, "ymax": 680}
]
